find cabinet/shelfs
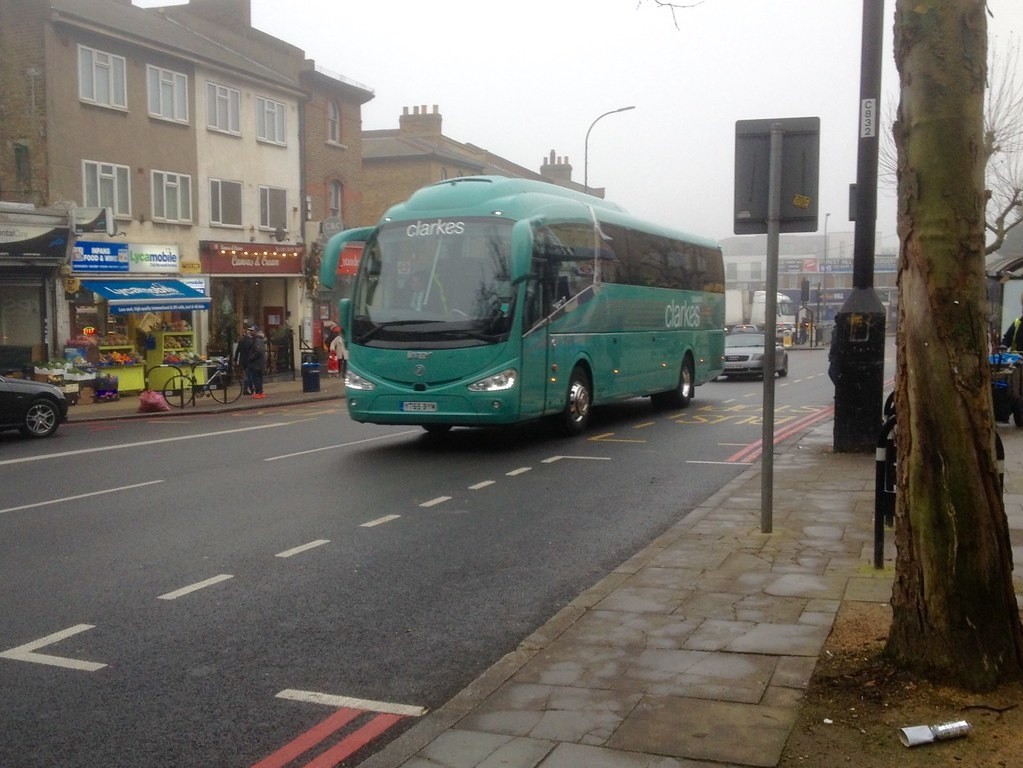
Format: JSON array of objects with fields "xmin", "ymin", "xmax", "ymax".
[
  {"xmin": 90, "ymin": 344, "xmax": 145, "ymax": 392},
  {"xmin": 147, "ymin": 330, "xmax": 208, "ymax": 391}
]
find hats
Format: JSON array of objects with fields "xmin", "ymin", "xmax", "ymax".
[{"xmin": 247, "ymin": 325, "xmax": 255, "ymax": 331}]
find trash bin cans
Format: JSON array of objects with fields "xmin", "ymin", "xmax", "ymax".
[{"xmin": 302, "ymin": 362, "xmax": 321, "ymax": 392}]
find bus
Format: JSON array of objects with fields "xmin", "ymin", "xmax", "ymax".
[{"xmin": 317, "ymin": 174, "xmax": 728, "ymax": 437}]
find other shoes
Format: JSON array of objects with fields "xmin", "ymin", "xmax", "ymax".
[
  {"xmin": 251, "ymin": 393, "xmax": 265, "ymax": 398},
  {"xmin": 250, "ymin": 386, "xmax": 254, "ymax": 391},
  {"xmin": 243, "ymin": 389, "xmax": 248, "ymax": 395}
]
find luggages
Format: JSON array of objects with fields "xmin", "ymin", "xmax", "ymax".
[{"xmin": 328, "ymin": 353, "xmax": 340, "ymax": 379}]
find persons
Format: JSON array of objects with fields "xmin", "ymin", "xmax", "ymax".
[
  {"xmin": 410, "ymin": 273, "xmax": 432, "ymax": 311},
  {"xmin": 234, "ymin": 327, "xmax": 267, "ymax": 398},
  {"xmin": 1001, "ymin": 316, "xmax": 1023, "ymax": 355},
  {"xmin": 325, "ymin": 323, "xmax": 349, "ymax": 378}
]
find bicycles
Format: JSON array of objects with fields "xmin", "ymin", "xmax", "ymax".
[{"xmin": 162, "ymin": 360, "xmax": 243, "ymax": 409}]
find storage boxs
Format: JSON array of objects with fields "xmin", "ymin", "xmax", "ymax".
[{"xmin": 137, "ymin": 313, "xmax": 161, "ymax": 333}]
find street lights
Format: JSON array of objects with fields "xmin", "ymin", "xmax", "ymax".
[
  {"xmin": 822, "ymin": 211, "xmax": 832, "ymax": 324},
  {"xmin": 582, "ymin": 103, "xmax": 639, "ymax": 196}
]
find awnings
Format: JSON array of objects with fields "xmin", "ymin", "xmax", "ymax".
[{"xmin": 81, "ymin": 278, "xmax": 213, "ymax": 314}]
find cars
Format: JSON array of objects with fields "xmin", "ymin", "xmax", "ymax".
[
  {"xmin": 709, "ymin": 334, "xmax": 789, "ymax": 383},
  {"xmin": 0, "ymin": 375, "xmax": 69, "ymax": 438}
]
